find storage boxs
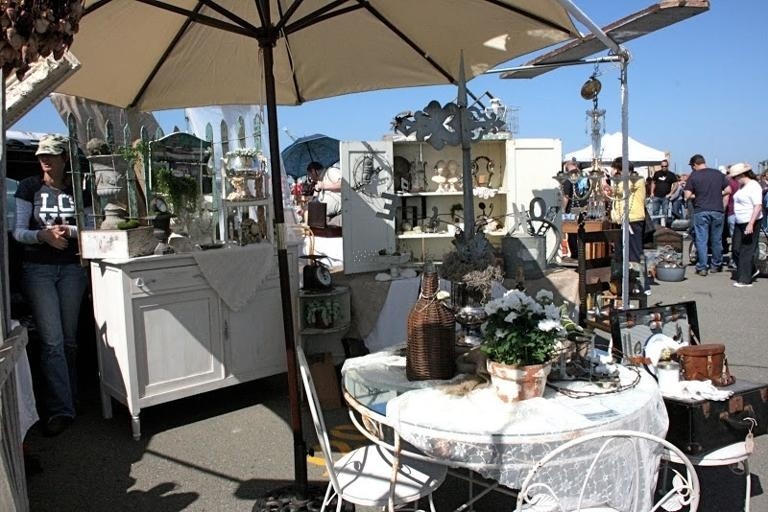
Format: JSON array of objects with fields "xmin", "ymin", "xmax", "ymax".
[{"xmin": 656, "ymin": 374, "xmax": 767, "ymax": 456}]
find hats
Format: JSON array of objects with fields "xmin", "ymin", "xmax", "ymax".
[
  {"xmin": 718, "ymin": 165, "xmax": 728, "ymax": 177},
  {"xmin": 34, "ymin": 133, "xmax": 71, "ymax": 158},
  {"xmin": 725, "ymin": 162, "xmax": 755, "ymax": 178}
]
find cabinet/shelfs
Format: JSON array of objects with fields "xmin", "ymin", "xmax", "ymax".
[
  {"xmin": 339, "ymin": 135, "xmax": 564, "ymax": 276},
  {"xmin": 87, "ymin": 245, "xmax": 304, "ymax": 441}
]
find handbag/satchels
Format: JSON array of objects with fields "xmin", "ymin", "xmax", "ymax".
[{"xmin": 675, "ymin": 340, "xmax": 737, "ymax": 389}]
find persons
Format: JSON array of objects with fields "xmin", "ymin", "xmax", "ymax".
[
  {"xmin": 13, "ymin": 134, "xmax": 95, "ymax": 435},
  {"xmin": 683, "ymin": 154, "xmax": 732, "ymax": 276},
  {"xmin": 611, "ymin": 158, "xmax": 646, "ymax": 263},
  {"xmin": 645, "ymin": 176, "xmax": 654, "ymax": 198},
  {"xmin": 649, "ymin": 159, "xmax": 679, "ymax": 228},
  {"xmin": 723, "ymin": 169, "xmax": 743, "ymax": 269},
  {"xmin": 307, "ymin": 161, "xmax": 342, "ymax": 229},
  {"xmin": 726, "ymin": 162, "xmax": 764, "ymax": 288},
  {"xmin": 562, "ymin": 162, "xmax": 587, "ymax": 262}
]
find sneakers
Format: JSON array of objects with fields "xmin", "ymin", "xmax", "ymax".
[
  {"xmin": 733, "ymin": 281, "xmax": 754, "ymax": 289},
  {"xmin": 696, "ymin": 270, "xmax": 708, "ymax": 276},
  {"xmin": 709, "ymin": 268, "xmax": 720, "ymax": 273},
  {"xmin": 752, "ymin": 269, "xmax": 761, "ymax": 279}
]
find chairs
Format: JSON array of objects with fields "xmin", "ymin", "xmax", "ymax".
[
  {"xmin": 662, "ymin": 423, "xmax": 755, "ymax": 512},
  {"xmin": 577, "ymin": 227, "xmax": 650, "ymax": 363},
  {"xmin": 296, "ymin": 345, "xmax": 448, "ymax": 511},
  {"xmin": 516, "ymin": 430, "xmax": 700, "ymax": 512}
]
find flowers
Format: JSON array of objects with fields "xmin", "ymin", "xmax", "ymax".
[{"xmin": 474, "ymin": 287, "xmax": 587, "ymax": 368}]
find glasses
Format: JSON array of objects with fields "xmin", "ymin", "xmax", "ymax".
[{"xmin": 660, "ymin": 165, "xmax": 668, "ymax": 168}]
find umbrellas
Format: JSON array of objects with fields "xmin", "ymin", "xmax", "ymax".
[
  {"xmin": 48, "ymin": 0, "xmax": 585, "ymax": 512},
  {"xmin": 562, "ymin": 131, "xmax": 671, "ymax": 185},
  {"xmin": 279, "ymin": 133, "xmax": 341, "ymax": 180}
]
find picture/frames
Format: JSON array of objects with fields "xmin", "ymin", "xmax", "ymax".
[{"xmin": 608, "ymin": 300, "xmax": 701, "ymax": 368}]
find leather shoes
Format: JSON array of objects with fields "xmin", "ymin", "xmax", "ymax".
[{"xmin": 44, "ymin": 413, "xmax": 69, "ymax": 439}]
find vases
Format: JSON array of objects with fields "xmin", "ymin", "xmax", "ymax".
[{"xmin": 484, "ymin": 358, "xmax": 554, "ymax": 404}]
